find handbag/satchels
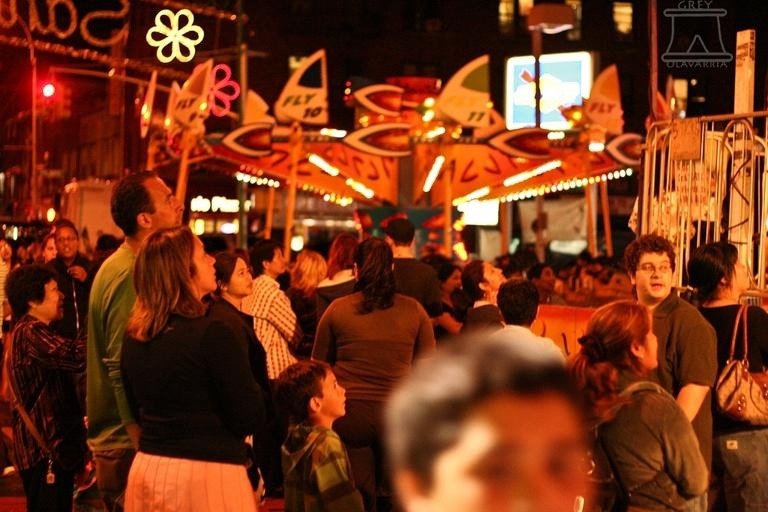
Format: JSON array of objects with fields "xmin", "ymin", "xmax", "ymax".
[{"xmin": 715, "ymin": 303, "xmax": 767, "ymax": 427}]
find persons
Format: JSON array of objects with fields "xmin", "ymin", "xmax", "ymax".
[{"xmin": 0, "ymin": 171, "xmax": 768, "ymax": 512}]
